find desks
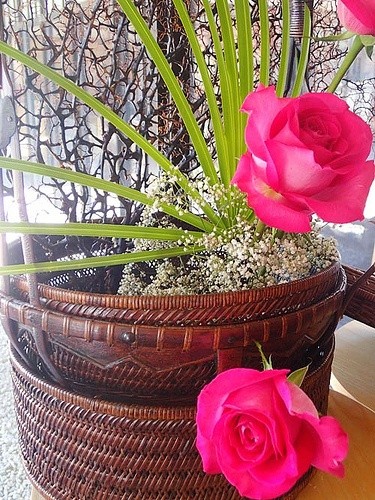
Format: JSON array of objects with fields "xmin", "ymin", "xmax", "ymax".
[{"xmin": 293, "ymin": 389, "xmax": 375, "ymax": 500}]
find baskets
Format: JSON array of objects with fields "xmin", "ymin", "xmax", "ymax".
[{"xmin": 3, "ymin": 215, "xmax": 345, "ymax": 500}]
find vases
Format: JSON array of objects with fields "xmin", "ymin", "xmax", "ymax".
[{"xmin": 0, "ymin": 214, "xmax": 347, "ymax": 500}]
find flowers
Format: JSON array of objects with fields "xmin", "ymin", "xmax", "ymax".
[
  {"xmin": 195, "ymin": 338, "xmax": 350, "ymax": 500},
  {"xmin": 0, "ymin": 0, "xmax": 375, "ymax": 296}
]
